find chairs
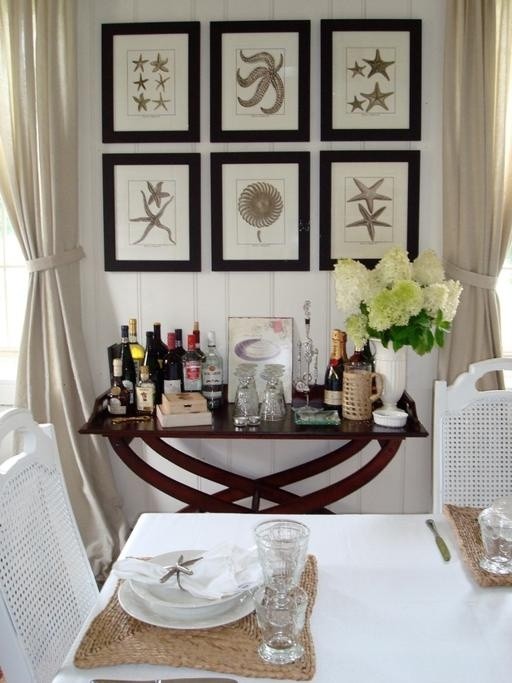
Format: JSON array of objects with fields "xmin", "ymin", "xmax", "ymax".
[
  {"xmin": 0, "ymin": 406, "xmax": 104, "ymax": 681},
  {"xmin": 427, "ymin": 354, "xmax": 510, "ymax": 513}
]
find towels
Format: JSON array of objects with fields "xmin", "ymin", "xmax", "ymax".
[{"xmin": 109, "ymin": 539, "xmax": 268, "ymax": 601}]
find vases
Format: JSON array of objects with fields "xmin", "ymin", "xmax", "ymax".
[{"xmin": 367, "ymin": 331, "xmax": 413, "ymax": 428}]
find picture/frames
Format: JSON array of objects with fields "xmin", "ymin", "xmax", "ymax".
[
  {"xmin": 208, "ymin": 149, "xmax": 312, "ymax": 274},
  {"xmin": 316, "ymin": 148, "xmax": 421, "ymax": 271},
  {"xmin": 100, "ymin": 151, "xmax": 202, "ymax": 274},
  {"xmin": 317, "ymin": 14, "xmax": 423, "ymax": 145},
  {"xmin": 97, "ymin": 18, "xmax": 201, "ymax": 147},
  {"xmin": 204, "ymin": 19, "xmax": 313, "ymax": 143}
]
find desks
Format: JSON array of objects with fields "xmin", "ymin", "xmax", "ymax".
[{"xmin": 77, "ymin": 383, "xmax": 430, "ymax": 514}]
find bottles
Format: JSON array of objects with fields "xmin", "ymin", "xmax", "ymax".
[
  {"xmin": 105, "ymin": 358, "xmax": 129, "ymax": 415},
  {"xmin": 136, "ymin": 366, "xmax": 155, "ymax": 414},
  {"xmin": 324, "ymin": 328, "xmax": 348, "ymax": 410},
  {"xmin": 120, "ymin": 318, "xmax": 224, "ymax": 409},
  {"xmin": 477, "ymin": 497, "xmax": 512, "ymax": 576},
  {"xmin": 348, "ymin": 342, "xmax": 371, "ymax": 371}
]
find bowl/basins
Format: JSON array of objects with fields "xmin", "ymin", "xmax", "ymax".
[
  {"xmin": 129, "ymin": 549, "xmax": 262, "ymax": 614},
  {"xmin": 372, "ymin": 408, "xmax": 408, "ymax": 427}
]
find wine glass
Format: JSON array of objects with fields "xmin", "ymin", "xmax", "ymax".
[
  {"xmin": 256, "ymin": 517, "xmax": 312, "ymax": 626},
  {"xmin": 231, "ymin": 363, "xmax": 287, "ymax": 427}
]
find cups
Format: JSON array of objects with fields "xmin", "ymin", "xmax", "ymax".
[
  {"xmin": 343, "ymin": 361, "xmax": 384, "ymax": 421},
  {"xmin": 253, "ymin": 579, "xmax": 308, "ymax": 664}
]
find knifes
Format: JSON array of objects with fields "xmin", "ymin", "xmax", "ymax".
[
  {"xmin": 425, "ymin": 518, "xmax": 450, "ymax": 561},
  {"xmin": 89, "ymin": 676, "xmax": 237, "ymax": 682}
]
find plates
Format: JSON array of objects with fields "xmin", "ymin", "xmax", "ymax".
[{"xmin": 117, "ymin": 579, "xmax": 255, "ymax": 631}]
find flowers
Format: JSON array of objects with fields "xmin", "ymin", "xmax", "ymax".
[{"xmin": 330, "ymin": 244, "xmax": 466, "ymax": 357}]
